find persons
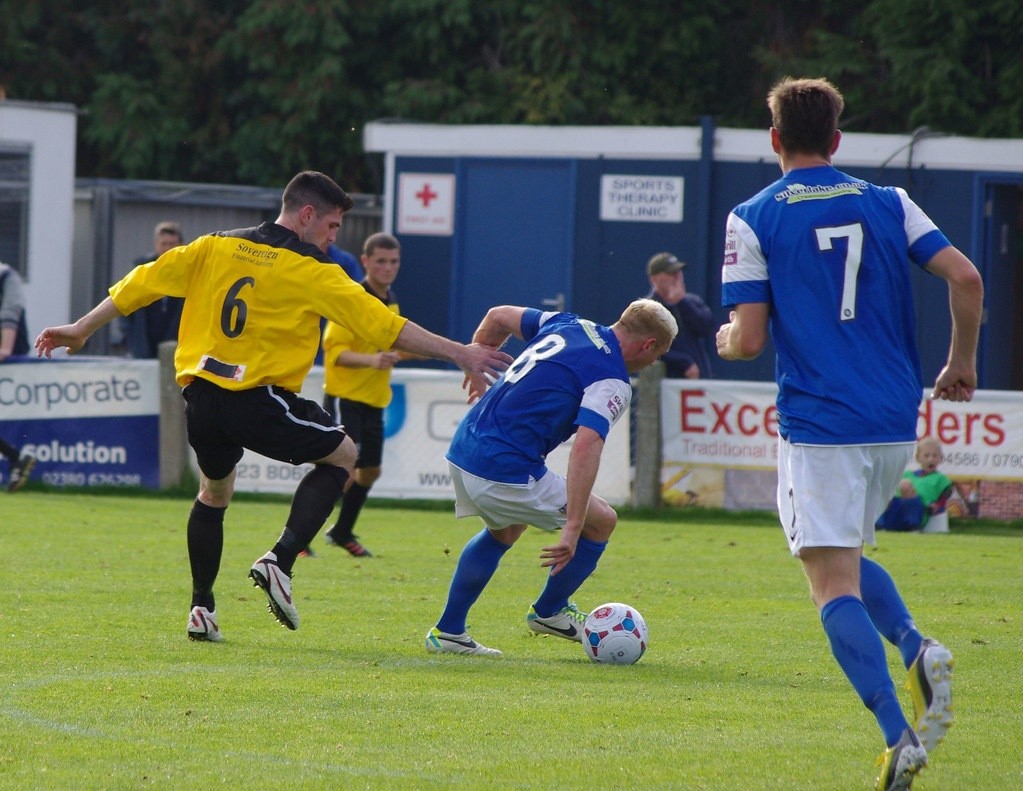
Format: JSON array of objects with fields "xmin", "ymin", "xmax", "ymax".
[
  {"xmin": 874, "ymin": 438, "xmax": 953, "ymax": 530},
  {"xmin": 35, "ymin": 171, "xmax": 498, "ymax": 624},
  {"xmin": 117, "ymin": 222, "xmax": 185, "ymax": 359},
  {"xmin": 0, "ymin": 439, "xmax": 34, "ymax": 492},
  {"xmin": 314, "ymin": 242, "xmax": 364, "ymax": 365},
  {"xmin": 646, "ymin": 253, "xmax": 716, "ymax": 379},
  {"xmin": 717, "ymin": 77, "xmax": 984, "ymax": 791},
  {"xmin": 297, "ymin": 231, "xmax": 401, "ymax": 555},
  {"xmin": 425, "ymin": 298, "xmax": 679, "ymax": 660},
  {"xmin": 0, "ymin": 262, "xmax": 31, "ymax": 362}
]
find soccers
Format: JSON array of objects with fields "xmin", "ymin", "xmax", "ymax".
[{"xmin": 581, "ymin": 601, "xmax": 648, "ymax": 665}]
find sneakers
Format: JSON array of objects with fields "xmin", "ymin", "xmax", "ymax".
[
  {"xmin": 300, "ymin": 547, "xmax": 311, "ymax": 557},
  {"xmin": 904, "ymin": 637, "xmax": 954, "ymax": 753},
  {"xmin": 248, "ymin": 551, "xmax": 298, "ymax": 631},
  {"xmin": 326, "ymin": 524, "xmax": 372, "ymax": 558},
  {"xmin": 527, "ymin": 603, "xmax": 589, "ymax": 644},
  {"xmin": 425, "ymin": 627, "xmax": 502, "ymax": 655},
  {"xmin": 873, "ymin": 728, "xmax": 929, "ymax": 791},
  {"xmin": 187, "ymin": 605, "xmax": 222, "ymax": 642}
]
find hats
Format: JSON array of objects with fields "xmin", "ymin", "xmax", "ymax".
[{"xmin": 648, "ymin": 253, "xmax": 687, "ymax": 276}]
